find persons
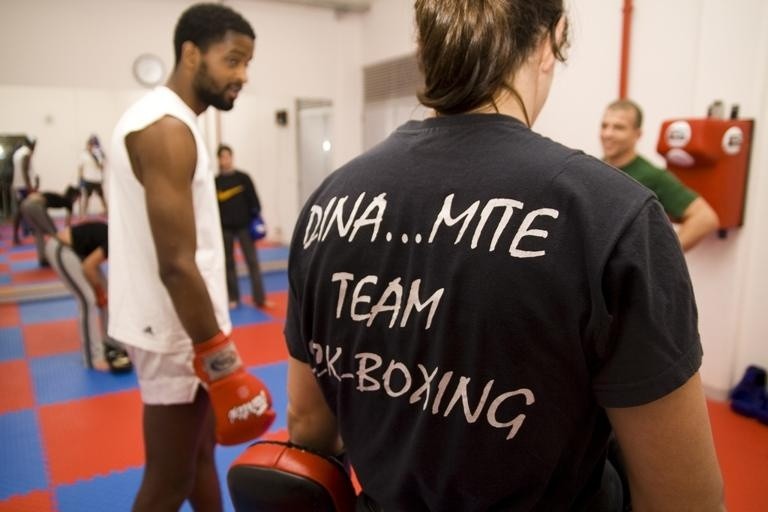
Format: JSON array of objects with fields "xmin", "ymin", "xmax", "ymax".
[
  {"xmin": 599, "ymin": 98, "xmax": 720, "ymax": 251},
  {"xmin": 214, "ymin": 143, "xmax": 276, "ymax": 309},
  {"xmin": 105, "ymin": 2, "xmax": 275, "ymax": 510},
  {"xmin": 281, "ymin": 0, "xmax": 725, "ymax": 512},
  {"xmin": 19, "ymin": 186, "xmax": 80, "ymax": 266},
  {"xmin": 77, "ymin": 134, "xmax": 109, "ymax": 217},
  {"xmin": 9, "ymin": 137, "xmax": 35, "ymax": 247},
  {"xmin": 45, "ymin": 221, "xmax": 110, "ymax": 373}
]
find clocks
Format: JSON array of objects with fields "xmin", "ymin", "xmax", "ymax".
[{"xmin": 131, "ymin": 53, "xmax": 167, "ymax": 89}]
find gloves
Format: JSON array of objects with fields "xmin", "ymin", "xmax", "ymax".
[
  {"xmin": 190, "ymin": 329, "xmax": 279, "ymax": 446},
  {"xmin": 250, "ymin": 218, "xmax": 268, "ymax": 242}
]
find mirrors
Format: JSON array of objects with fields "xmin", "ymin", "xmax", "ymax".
[{"xmin": 0, "ymin": 1, "xmax": 220, "ymax": 296}]
